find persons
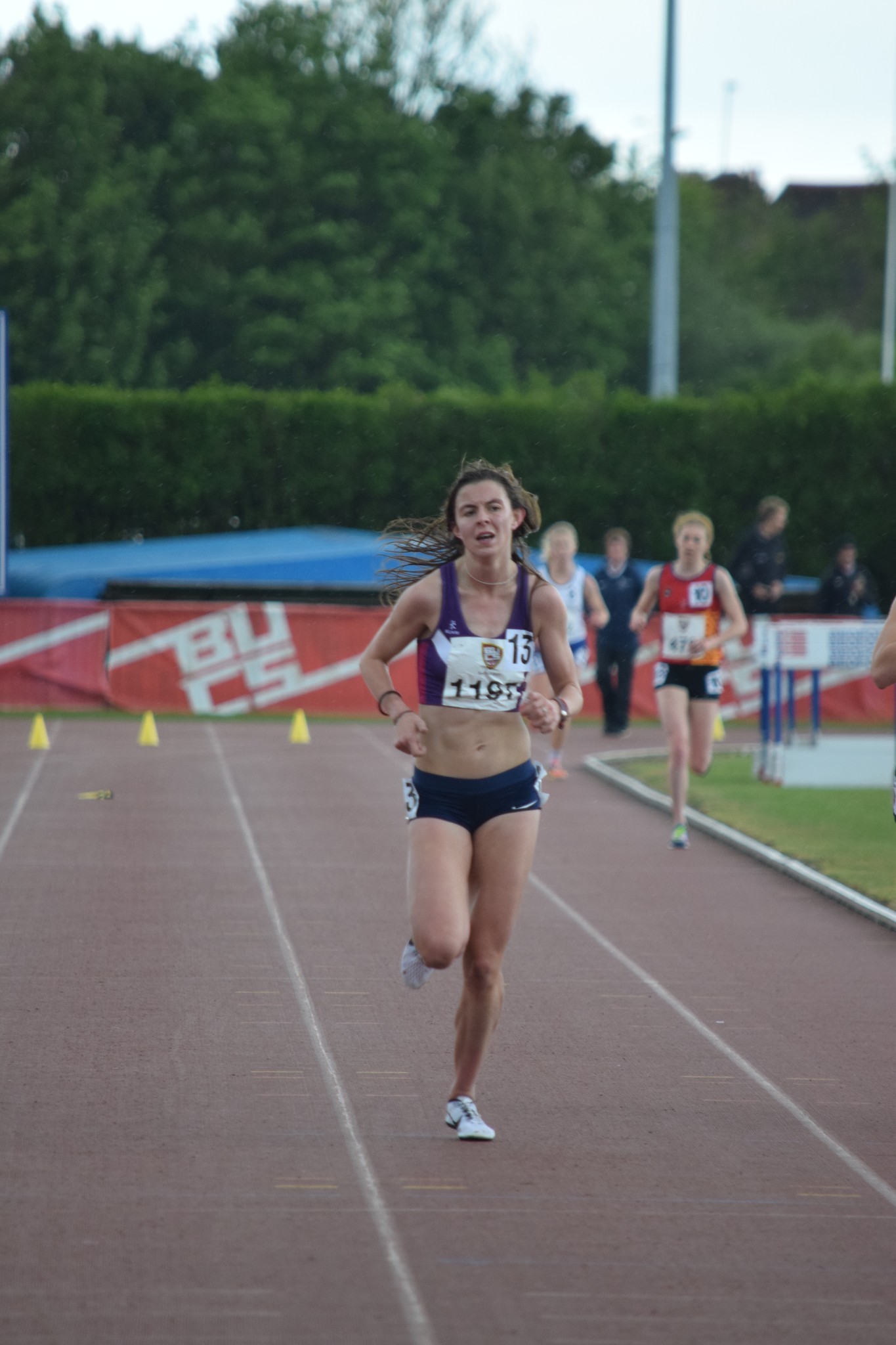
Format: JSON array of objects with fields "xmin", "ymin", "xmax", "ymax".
[
  {"xmin": 869, "ymin": 596, "xmax": 896, "ymax": 689},
  {"xmin": 358, "ymin": 457, "xmax": 586, "ymax": 1142},
  {"xmin": 585, "ymin": 527, "xmax": 644, "ymax": 737},
  {"xmin": 530, "ymin": 521, "xmax": 612, "ymax": 780},
  {"xmin": 729, "ymin": 495, "xmax": 797, "ymax": 616},
  {"xmin": 813, "ymin": 547, "xmax": 867, "ymax": 617},
  {"xmin": 629, "ymin": 508, "xmax": 748, "ymax": 848}
]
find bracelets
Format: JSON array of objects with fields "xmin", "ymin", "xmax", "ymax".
[
  {"xmin": 377, "ymin": 690, "xmax": 402, "ymax": 716},
  {"xmin": 391, "ymin": 709, "xmax": 414, "ymax": 725},
  {"xmin": 549, "ymin": 695, "xmax": 572, "ymax": 732}
]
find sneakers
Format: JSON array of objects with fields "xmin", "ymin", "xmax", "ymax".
[
  {"xmin": 400, "ymin": 937, "xmax": 435, "ymax": 989},
  {"xmin": 445, "ymin": 1095, "xmax": 495, "ymax": 1141}
]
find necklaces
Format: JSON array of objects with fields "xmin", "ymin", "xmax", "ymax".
[{"xmin": 463, "ymin": 561, "xmax": 520, "ymax": 585}]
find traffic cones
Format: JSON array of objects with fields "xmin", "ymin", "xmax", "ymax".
[
  {"xmin": 710, "ymin": 711, "xmax": 728, "ymax": 745},
  {"xmin": 289, "ymin": 707, "xmax": 312, "ymax": 743},
  {"xmin": 25, "ymin": 712, "xmax": 51, "ymax": 752},
  {"xmin": 136, "ymin": 709, "xmax": 162, "ymax": 748}
]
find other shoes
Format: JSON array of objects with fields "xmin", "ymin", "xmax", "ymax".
[
  {"xmin": 547, "ymin": 767, "xmax": 568, "ymax": 781},
  {"xmin": 669, "ymin": 823, "xmax": 690, "ymax": 849}
]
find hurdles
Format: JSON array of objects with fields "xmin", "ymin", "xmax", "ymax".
[{"xmin": 751, "ymin": 651, "xmax": 896, "ymax": 788}]
